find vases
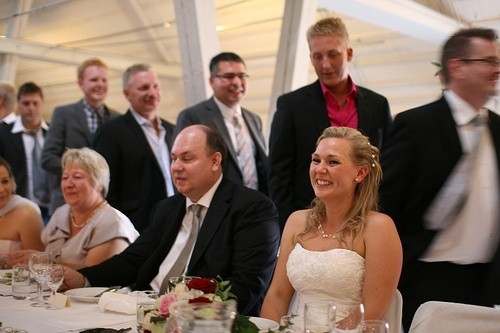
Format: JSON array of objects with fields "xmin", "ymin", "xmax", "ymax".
[{"xmin": 190, "ymin": 318, "xmax": 232, "ymax": 333}]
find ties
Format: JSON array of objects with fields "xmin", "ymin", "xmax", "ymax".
[
  {"xmin": 24, "ymin": 127, "xmax": 50, "ymax": 204},
  {"xmin": 421, "ymin": 115, "xmax": 489, "ymax": 229},
  {"xmin": 231, "ymin": 111, "xmax": 259, "ymax": 191},
  {"xmin": 94, "ymin": 108, "xmax": 106, "ymax": 129},
  {"xmin": 158, "ymin": 204, "xmax": 204, "ymax": 299}
]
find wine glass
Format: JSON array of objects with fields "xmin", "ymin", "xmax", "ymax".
[
  {"xmin": 27, "ymin": 250, "xmax": 55, "ymax": 307},
  {"xmin": 45, "ymin": 264, "xmax": 65, "ymax": 310}
]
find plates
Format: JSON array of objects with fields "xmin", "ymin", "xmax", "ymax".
[
  {"xmin": 64, "ymin": 286, "xmax": 123, "ymax": 304},
  {"xmin": 0, "ymin": 269, "xmax": 36, "ymax": 283}
]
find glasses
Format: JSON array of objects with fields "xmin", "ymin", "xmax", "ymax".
[
  {"xmin": 211, "ymin": 71, "xmax": 250, "ymax": 82},
  {"xmin": 460, "ymin": 55, "xmax": 500, "ymax": 67}
]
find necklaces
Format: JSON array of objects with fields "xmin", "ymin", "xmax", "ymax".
[
  {"xmin": 318, "ymin": 220, "xmax": 336, "ymax": 238},
  {"xmin": 0, "ymin": 216, "xmax": 4, "ymax": 222},
  {"xmin": 70, "ymin": 200, "xmax": 107, "ymax": 228}
]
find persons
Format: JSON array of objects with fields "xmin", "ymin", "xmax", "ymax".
[
  {"xmin": 259, "ymin": 126, "xmax": 402, "ymax": 333},
  {"xmin": 379, "ymin": 28, "xmax": 500, "ymax": 333},
  {"xmin": 6, "ymin": 147, "xmax": 140, "ymax": 271},
  {"xmin": 0, "ymin": 156, "xmax": 46, "ymax": 258},
  {"xmin": 266, "ymin": 17, "xmax": 393, "ymax": 242},
  {"xmin": 0, "ymin": 82, "xmax": 50, "ymax": 226},
  {"xmin": 167, "ymin": 52, "xmax": 268, "ymax": 196},
  {"xmin": 90, "ymin": 63, "xmax": 176, "ymax": 235},
  {"xmin": 50, "ymin": 125, "xmax": 280, "ymax": 315},
  {"xmin": 39, "ymin": 59, "xmax": 126, "ymax": 226}
]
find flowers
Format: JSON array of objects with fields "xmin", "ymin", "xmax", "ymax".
[{"xmin": 139, "ymin": 275, "xmax": 238, "ymax": 333}]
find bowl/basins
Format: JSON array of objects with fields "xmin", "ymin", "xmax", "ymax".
[{"xmin": 249, "ymin": 316, "xmax": 280, "ymax": 333}]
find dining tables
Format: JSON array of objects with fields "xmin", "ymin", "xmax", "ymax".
[{"xmin": 0, "ymin": 273, "xmax": 143, "ymax": 333}]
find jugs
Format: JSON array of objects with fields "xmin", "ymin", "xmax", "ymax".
[{"xmin": 161, "ymin": 300, "xmax": 236, "ymax": 333}]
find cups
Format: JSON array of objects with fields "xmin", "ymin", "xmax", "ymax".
[
  {"xmin": 359, "ymin": 319, "xmax": 390, "ymax": 333},
  {"xmin": 136, "ymin": 290, "xmax": 159, "ymax": 333},
  {"xmin": 11, "ymin": 264, "xmax": 31, "ymax": 300},
  {"xmin": 303, "ymin": 301, "xmax": 337, "ymax": 333},
  {"xmin": 328, "ymin": 300, "xmax": 365, "ymax": 333}
]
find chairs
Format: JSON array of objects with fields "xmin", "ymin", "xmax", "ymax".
[
  {"xmin": 408, "ymin": 299, "xmax": 500, "ymax": 333},
  {"xmin": 381, "ymin": 288, "xmax": 404, "ymax": 333}
]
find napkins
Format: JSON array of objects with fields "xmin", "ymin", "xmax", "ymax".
[{"xmin": 95, "ymin": 288, "xmax": 136, "ymax": 316}]
